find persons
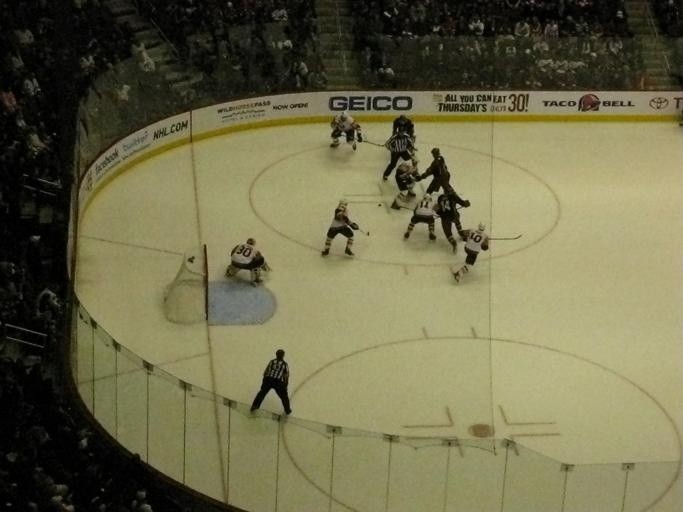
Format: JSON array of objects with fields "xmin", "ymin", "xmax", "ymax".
[
  {"xmin": 223, "ymin": 236, "xmax": 270, "ymax": 288},
  {"xmin": 451, "ymin": 222, "xmax": 489, "ymax": 287},
  {"xmin": 417, "ymin": 146, "xmax": 470, "ymax": 210},
  {"xmin": 248, "ymin": 350, "xmax": 293, "ymax": 416},
  {"xmin": 392, "ymin": 115, "xmax": 416, "ymax": 152},
  {"xmin": 1, "ymin": 130, "xmax": 191, "ymax": 511},
  {"xmin": 390, "ymin": 156, "xmax": 421, "ymax": 213},
  {"xmin": 402, "ymin": 193, "xmax": 439, "ymax": 243},
  {"xmin": 329, "ymin": 110, "xmax": 363, "ymax": 151},
  {"xmin": 382, "ymin": 127, "xmax": 419, "ymax": 182},
  {"xmin": 434, "ymin": 187, "xmax": 467, "ymax": 256},
  {"xmin": 319, "ymin": 200, "xmax": 361, "ymax": 257},
  {"xmin": 1, "ymin": 0, "xmax": 683, "ymax": 134}
]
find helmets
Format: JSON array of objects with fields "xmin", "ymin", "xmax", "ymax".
[
  {"xmin": 340, "ymin": 108, "xmax": 408, "ymax": 140},
  {"xmin": 246, "ymin": 237, "xmax": 256, "ymax": 246},
  {"xmin": 337, "ymin": 197, "xmax": 350, "ymax": 206},
  {"xmin": 430, "ymin": 144, "xmax": 487, "ymax": 232}
]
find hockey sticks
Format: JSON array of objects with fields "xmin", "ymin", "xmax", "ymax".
[
  {"xmin": 340, "ymin": 134, "xmax": 385, "ymax": 146},
  {"xmin": 489, "ymin": 234, "xmax": 522, "ymax": 240},
  {"xmin": 396, "ymin": 206, "xmax": 414, "ymax": 210},
  {"xmin": 358, "ymin": 228, "xmax": 370, "ymax": 236}
]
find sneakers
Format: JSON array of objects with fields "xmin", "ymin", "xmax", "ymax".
[
  {"xmin": 319, "ymin": 247, "xmax": 355, "ymax": 256},
  {"xmin": 403, "ymin": 230, "xmax": 436, "ymax": 240},
  {"xmin": 329, "ymin": 140, "xmax": 356, "ymax": 151}
]
